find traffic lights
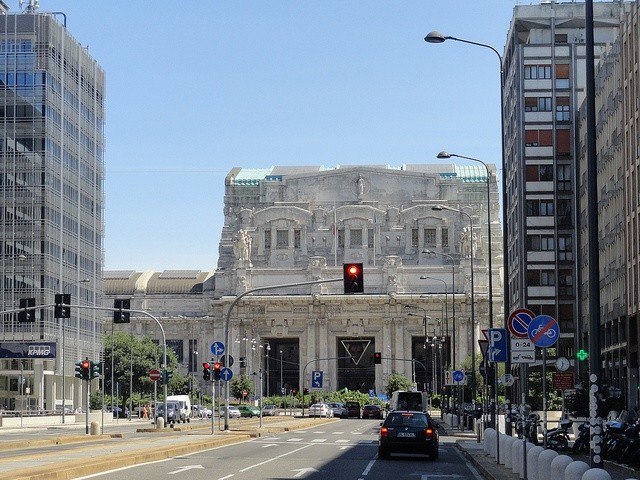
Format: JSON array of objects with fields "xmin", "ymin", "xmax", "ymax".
[
  {"xmin": 292, "ymin": 388, "xmax": 297, "ymax": 397},
  {"xmin": 160, "ymin": 369, "xmax": 173, "ymax": 385},
  {"xmin": 114, "ymin": 299, "xmax": 130, "ymax": 323},
  {"xmin": 374, "ymin": 352, "xmax": 381, "ymax": 364},
  {"xmin": 20, "ymin": 298, "xmax": 35, "ymax": 322},
  {"xmin": 214, "ymin": 363, "xmax": 220, "ymax": 380},
  {"xmin": 239, "ymin": 357, "xmax": 247, "ymax": 368},
  {"xmin": 55, "ymin": 294, "xmax": 70, "ymax": 318},
  {"xmin": 281, "ymin": 388, "xmax": 286, "ymax": 395},
  {"xmin": 344, "ymin": 264, "xmax": 363, "ymax": 293},
  {"xmin": 75, "ymin": 358, "xmax": 99, "ymax": 380},
  {"xmin": 303, "ymin": 388, "xmax": 309, "ymax": 395},
  {"xmin": 203, "ymin": 362, "xmax": 210, "ymax": 380}
]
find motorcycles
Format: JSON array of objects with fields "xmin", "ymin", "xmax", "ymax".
[{"xmin": 504, "ymin": 404, "xmax": 640, "ymax": 467}]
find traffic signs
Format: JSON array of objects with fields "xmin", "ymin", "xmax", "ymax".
[
  {"xmin": 312, "ymin": 371, "xmax": 323, "ymax": 388},
  {"xmin": 489, "ymin": 330, "xmax": 508, "ymax": 362}
]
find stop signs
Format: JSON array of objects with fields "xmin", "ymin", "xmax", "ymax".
[
  {"xmin": 242, "ymin": 390, "xmax": 248, "ymax": 396},
  {"xmin": 150, "ymin": 370, "xmax": 160, "ymax": 380}
]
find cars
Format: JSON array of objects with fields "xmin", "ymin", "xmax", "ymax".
[
  {"xmin": 328, "ymin": 403, "xmax": 347, "ymax": 418},
  {"xmin": 379, "ymin": 411, "xmax": 439, "ymax": 459},
  {"xmin": 237, "ymin": 406, "xmax": 261, "ymax": 418},
  {"xmin": 309, "ymin": 403, "xmax": 334, "ymax": 417},
  {"xmin": 190, "ymin": 405, "xmax": 212, "ymax": 418},
  {"xmin": 362, "ymin": 406, "xmax": 384, "ymax": 418},
  {"xmin": 261, "ymin": 405, "xmax": 280, "ymax": 417}
]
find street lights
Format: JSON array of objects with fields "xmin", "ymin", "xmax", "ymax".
[
  {"xmin": 234, "ymin": 338, "xmax": 271, "ymax": 396},
  {"xmin": 423, "ymin": 337, "xmax": 445, "ymax": 394},
  {"xmin": 432, "ymin": 203, "xmax": 478, "ymax": 401},
  {"xmin": 422, "ymin": 249, "xmax": 456, "ymax": 414},
  {"xmin": 129, "ymin": 308, "xmax": 152, "ymax": 421},
  {"xmin": 405, "ymin": 304, "xmax": 431, "ymax": 398},
  {"xmin": 112, "ymin": 297, "xmax": 135, "ymax": 423},
  {"xmin": 420, "ymin": 295, "xmax": 444, "ymax": 335},
  {"xmin": 437, "ymin": 151, "xmax": 500, "ymax": 464},
  {"xmin": 420, "ymin": 276, "xmax": 449, "ymax": 413},
  {"xmin": 62, "ymin": 278, "xmax": 90, "ymax": 423},
  {"xmin": 408, "ymin": 313, "xmax": 440, "ymax": 335}
]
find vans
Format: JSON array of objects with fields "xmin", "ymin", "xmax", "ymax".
[
  {"xmin": 167, "ymin": 395, "xmax": 193, "ymax": 423},
  {"xmin": 220, "ymin": 405, "xmax": 241, "ymax": 419},
  {"xmin": 389, "ymin": 391, "xmax": 427, "ymax": 411},
  {"xmin": 154, "ymin": 400, "xmax": 181, "ymax": 423},
  {"xmin": 346, "ymin": 402, "xmax": 360, "ymax": 418}
]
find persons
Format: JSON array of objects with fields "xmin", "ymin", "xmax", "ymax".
[
  {"xmin": 352, "ymin": 174, "xmax": 366, "ymax": 200},
  {"xmin": 459, "ymin": 226, "xmax": 478, "ymax": 259},
  {"xmin": 232, "ymin": 228, "xmax": 252, "ymax": 261}
]
